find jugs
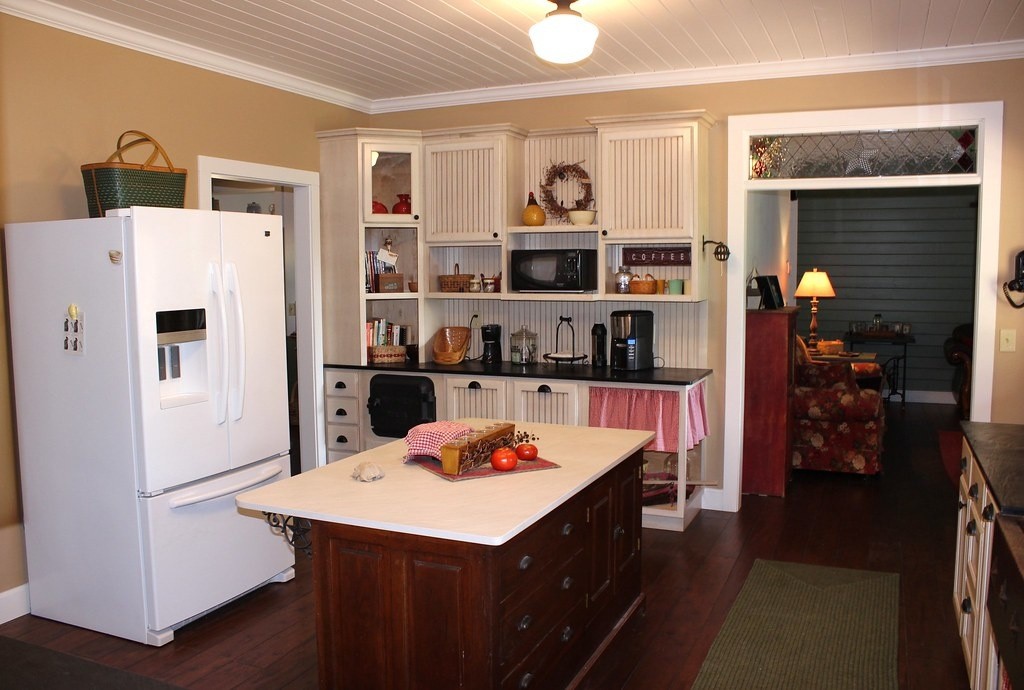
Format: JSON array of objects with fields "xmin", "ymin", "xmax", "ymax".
[{"xmin": 483, "ymin": 342, "xmax": 497, "ymax": 362}]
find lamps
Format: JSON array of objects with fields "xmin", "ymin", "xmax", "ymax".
[
  {"xmin": 529, "ymin": 0, "xmax": 599, "ymax": 65},
  {"xmin": 794, "ymin": 268, "xmax": 837, "ymax": 356},
  {"xmin": 703, "ymin": 234, "xmax": 731, "ymax": 278}
]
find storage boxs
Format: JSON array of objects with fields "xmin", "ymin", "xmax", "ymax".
[{"xmin": 374, "ymin": 273, "xmax": 404, "ymax": 293}]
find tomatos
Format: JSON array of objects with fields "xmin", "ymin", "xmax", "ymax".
[
  {"xmin": 490, "ymin": 447, "xmax": 518, "ymax": 471},
  {"xmin": 516, "ymin": 444, "xmax": 538, "ymax": 461}
]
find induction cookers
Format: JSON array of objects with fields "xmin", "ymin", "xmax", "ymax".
[{"xmin": 510, "ymin": 249, "xmax": 598, "ymax": 294}]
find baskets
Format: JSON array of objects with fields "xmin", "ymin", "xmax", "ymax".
[
  {"xmin": 372, "ymin": 345, "xmax": 407, "ymax": 364},
  {"xmin": 439, "ymin": 263, "xmax": 476, "ymax": 292},
  {"xmin": 375, "ymin": 273, "xmax": 404, "ymax": 293}
]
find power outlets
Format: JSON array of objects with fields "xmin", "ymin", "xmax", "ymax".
[{"xmin": 470, "ymin": 310, "xmax": 483, "ymax": 330}]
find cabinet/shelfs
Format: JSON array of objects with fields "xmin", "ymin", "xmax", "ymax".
[
  {"xmin": 952, "ymin": 438, "xmax": 1013, "ymax": 690},
  {"xmin": 324, "ymin": 371, "xmax": 706, "ymax": 689},
  {"xmin": 314, "ymin": 109, "xmax": 719, "ymax": 365},
  {"xmin": 741, "ymin": 304, "xmax": 802, "ymax": 499}
]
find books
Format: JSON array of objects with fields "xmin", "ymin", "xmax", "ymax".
[
  {"xmin": 366, "ymin": 251, "xmax": 397, "ymax": 292},
  {"xmin": 366, "ymin": 318, "xmax": 412, "ymax": 360}
]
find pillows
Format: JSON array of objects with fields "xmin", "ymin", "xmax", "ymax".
[{"xmin": 802, "ymin": 359, "xmax": 859, "ymax": 391}]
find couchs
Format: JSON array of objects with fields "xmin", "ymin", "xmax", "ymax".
[{"xmin": 794, "ymin": 334, "xmax": 885, "ymax": 482}]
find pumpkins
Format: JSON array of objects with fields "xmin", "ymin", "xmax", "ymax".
[{"xmin": 521, "ymin": 198, "xmax": 545, "ymax": 226}]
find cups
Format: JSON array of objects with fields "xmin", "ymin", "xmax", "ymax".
[
  {"xmin": 668, "ymin": 278, "xmax": 683, "ymax": 295},
  {"xmin": 655, "ymin": 279, "xmax": 668, "ymax": 295}
]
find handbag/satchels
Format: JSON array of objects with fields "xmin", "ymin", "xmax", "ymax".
[{"xmin": 80, "ymin": 131, "xmax": 187, "ymax": 217}]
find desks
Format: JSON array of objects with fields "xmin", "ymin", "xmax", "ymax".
[
  {"xmin": 842, "ymin": 332, "xmax": 915, "ymax": 412},
  {"xmin": 811, "ymin": 352, "xmax": 878, "ymax": 363}
]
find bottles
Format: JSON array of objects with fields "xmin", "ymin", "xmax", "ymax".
[
  {"xmin": 392, "ymin": 194, "xmax": 411, "ymax": 214},
  {"xmin": 590, "ymin": 321, "xmax": 608, "ymax": 368},
  {"xmin": 614, "ymin": 265, "xmax": 633, "ymax": 295},
  {"xmin": 509, "ymin": 324, "xmax": 538, "ymax": 366}
]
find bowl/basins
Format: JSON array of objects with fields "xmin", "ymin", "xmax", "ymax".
[{"xmin": 568, "ymin": 211, "xmax": 596, "ymax": 225}]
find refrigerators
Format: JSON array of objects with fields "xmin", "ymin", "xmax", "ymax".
[{"xmin": 2, "ymin": 204, "xmax": 300, "ymax": 649}]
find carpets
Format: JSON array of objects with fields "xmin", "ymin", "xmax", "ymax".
[
  {"xmin": 881, "ymin": 389, "xmax": 957, "ymax": 405},
  {"xmin": 690, "ymin": 559, "xmax": 901, "ymax": 690}
]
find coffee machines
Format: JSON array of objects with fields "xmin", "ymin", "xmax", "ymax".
[
  {"xmin": 481, "ymin": 324, "xmax": 502, "ymax": 364},
  {"xmin": 610, "ymin": 310, "xmax": 654, "ymax": 371}
]
